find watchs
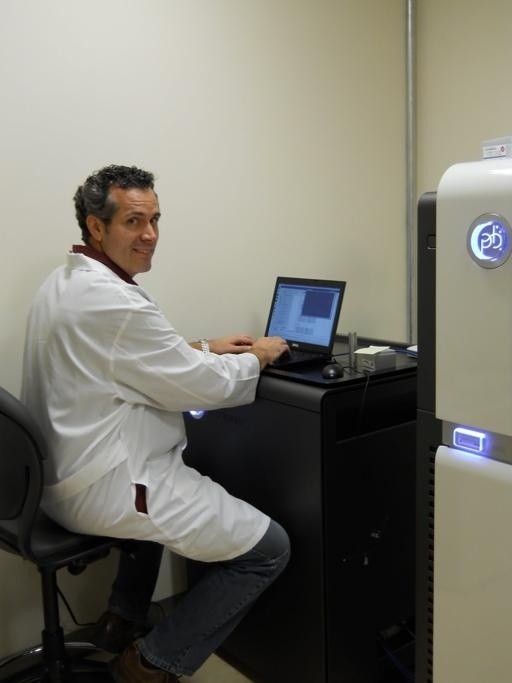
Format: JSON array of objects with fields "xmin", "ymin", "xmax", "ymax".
[{"xmin": 200, "ymin": 338, "xmax": 210, "ymax": 353}]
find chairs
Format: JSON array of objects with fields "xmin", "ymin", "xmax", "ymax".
[{"xmin": 0, "ymin": 384, "xmax": 140, "ymax": 683}]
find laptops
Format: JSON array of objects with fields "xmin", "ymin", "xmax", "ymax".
[{"xmin": 263, "ymin": 276, "xmax": 347, "ymax": 367}]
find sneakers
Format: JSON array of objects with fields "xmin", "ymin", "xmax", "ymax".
[{"xmin": 97, "ymin": 612, "xmax": 183, "ymax": 683}]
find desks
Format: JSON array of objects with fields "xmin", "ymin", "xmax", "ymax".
[{"xmin": 179, "ymin": 332, "xmax": 418, "ymax": 683}]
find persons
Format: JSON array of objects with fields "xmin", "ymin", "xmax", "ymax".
[{"xmin": 19, "ymin": 164, "xmax": 291, "ymax": 683}]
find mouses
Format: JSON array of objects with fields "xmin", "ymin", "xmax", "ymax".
[{"xmin": 322, "ymin": 363, "xmax": 344, "ymax": 379}]
